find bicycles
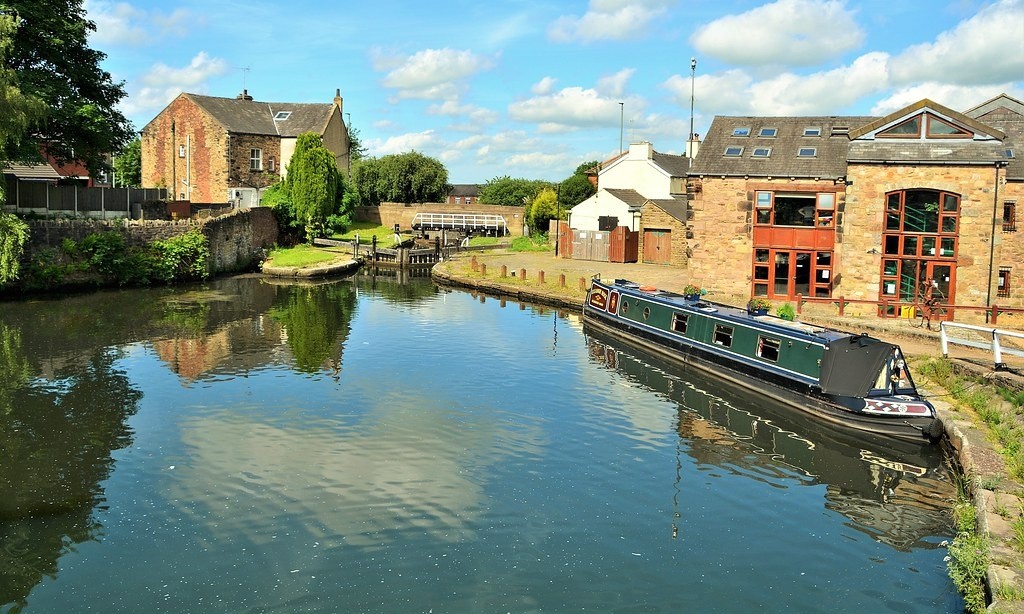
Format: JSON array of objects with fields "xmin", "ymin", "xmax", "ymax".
[{"xmin": 904, "ymin": 284, "xmax": 947, "ymax": 332}]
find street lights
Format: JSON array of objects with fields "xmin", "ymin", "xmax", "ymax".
[
  {"xmin": 689, "ymin": 56, "xmax": 697, "ymax": 169},
  {"xmin": 555, "ymin": 180, "xmax": 563, "ymax": 255},
  {"xmin": 618, "ymin": 102, "xmax": 624, "ymax": 158}
]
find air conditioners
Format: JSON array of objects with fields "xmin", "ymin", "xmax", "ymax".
[{"xmin": 232, "ymin": 190, "xmax": 242, "ymax": 199}]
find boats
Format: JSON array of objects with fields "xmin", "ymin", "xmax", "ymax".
[{"xmin": 581, "ymin": 270, "xmax": 946, "ymax": 445}]
